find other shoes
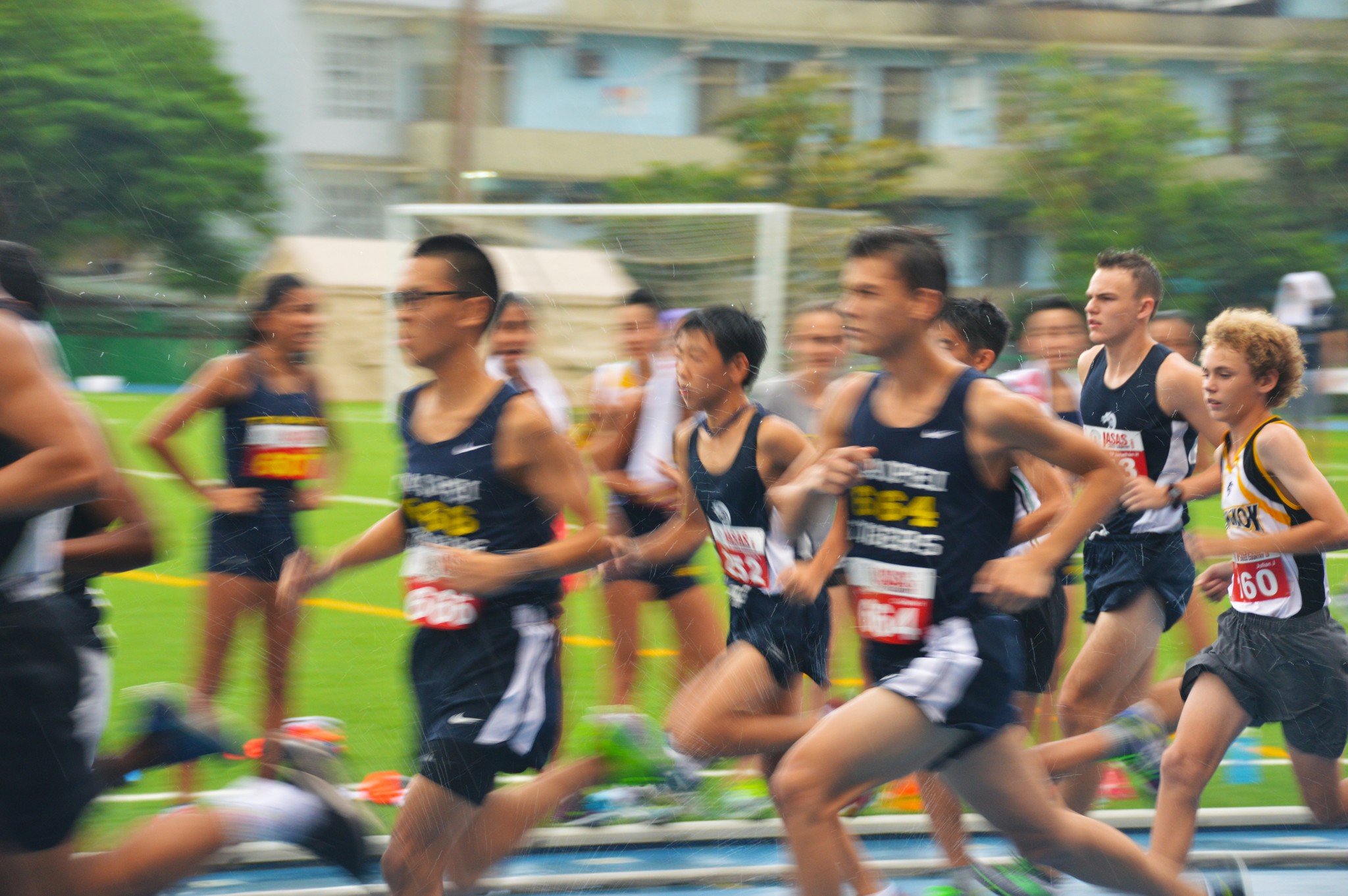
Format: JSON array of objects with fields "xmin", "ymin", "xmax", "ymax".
[
  {"xmin": 589, "ymin": 711, "xmax": 703, "ymax": 791},
  {"xmin": 276, "ymin": 765, "xmax": 368, "ymax": 884},
  {"xmin": 1114, "ymin": 700, "xmax": 1168, "ymax": 787},
  {"xmin": 1202, "ymin": 858, "xmax": 1248, "ymax": 896},
  {"xmin": 143, "ymin": 696, "xmax": 232, "ymax": 760}
]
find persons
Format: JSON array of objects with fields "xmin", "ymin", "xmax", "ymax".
[
  {"xmin": 1151, "ymin": 306, "xmax": 1348, "ymax": 875},
  {"xmin": 0, "ymin": 225, "xmax": 1262, "ymax": 895}
]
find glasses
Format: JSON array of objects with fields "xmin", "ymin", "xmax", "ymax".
[{"xmin": 388, "ymin": 288, "xmax": 459, "ymax": 310}]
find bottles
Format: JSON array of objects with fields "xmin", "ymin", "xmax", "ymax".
[{"xmin": 579, "ymin": 782, "xmax": 660, "ymax": 812}]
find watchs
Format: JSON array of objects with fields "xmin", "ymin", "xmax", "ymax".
[{"xmin": 1164, "ymin": 482, "xmax": 1183, "ymax": 511}]
